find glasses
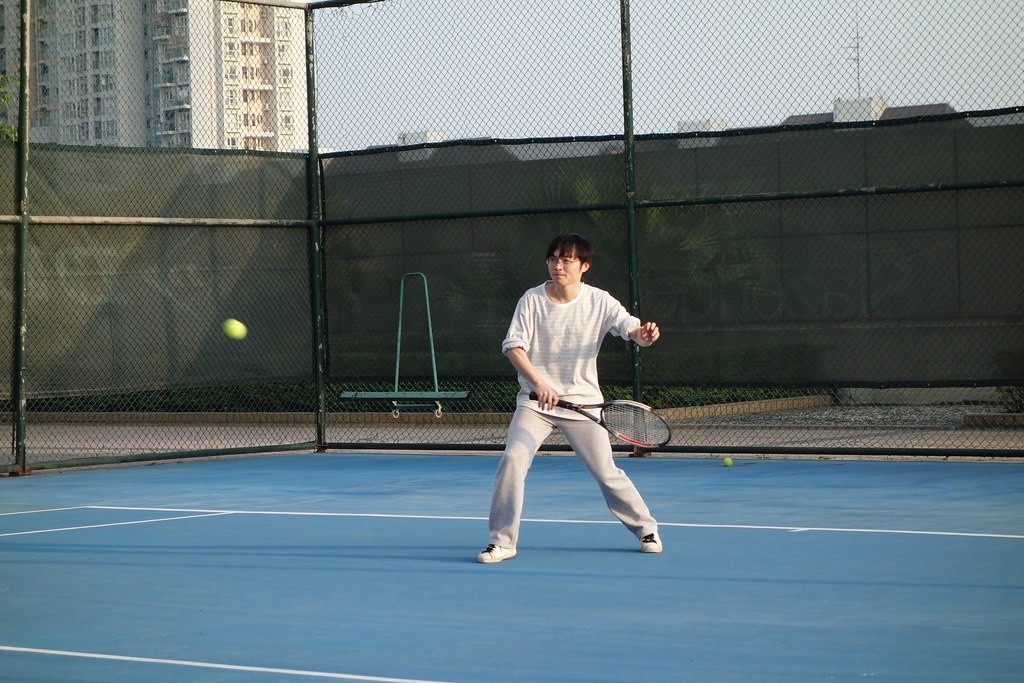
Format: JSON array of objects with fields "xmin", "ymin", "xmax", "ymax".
[{"xmin": 545, "ymin": 258, "xmax": 583, "ymax": 265}]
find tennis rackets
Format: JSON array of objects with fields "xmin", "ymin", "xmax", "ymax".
[{"xmin": 527, "ymin": 391, "xmax": 673, "ymax": 449}]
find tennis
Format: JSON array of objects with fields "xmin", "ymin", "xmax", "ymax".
[
  {"xmin": 222, "ymin": 319, "xmax": 248, "ymax": 340},
  {"xmin": 723, "ymin": 457, "xmax": 732, "ymax": 467}
]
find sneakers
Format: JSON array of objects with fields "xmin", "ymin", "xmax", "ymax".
[
  {"xmin": 639, "ymin": 532, "xmax": 663, "ymax": 553},
  {"xmin": 477, "ymin": 544, "xmax": 517, "ymax": 563}
]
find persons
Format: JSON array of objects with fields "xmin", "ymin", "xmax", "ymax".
[{"xmin": 476, "ymin": 232, "xmax": 663, "ymax": 563}]
